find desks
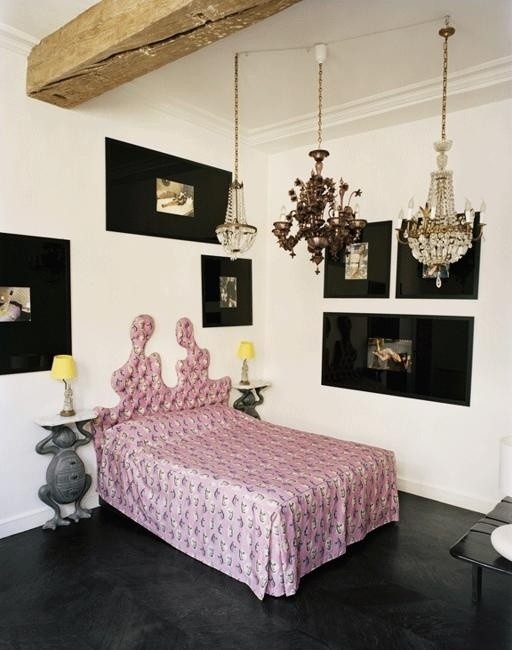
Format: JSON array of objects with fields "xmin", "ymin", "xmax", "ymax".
[
  {"xmin": 230, "ymin": 380, "xmax": 272, "ymax": 421},
  {"xmin": 32, "ymin": 411, "xmax": 97, "ymax": 531}
]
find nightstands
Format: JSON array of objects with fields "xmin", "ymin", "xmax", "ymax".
[{"xmin": 90, "ymin": 314, "xmax": 400, "ymax": 602}]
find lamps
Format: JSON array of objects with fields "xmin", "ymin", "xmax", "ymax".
[
  {"xmin": 395, "ymin": 18, "xmax": 486, "ymax": 289},
  {"xmin": 272, "ymin": 43, "xmax": 366, "ymax": 276},
  {"xmin": 51, "ymin": 355, "xmax": 77, "ymax": 417},
  {"xmin": 237, "ymin": 341, "xmax": 256, "ymax": 386},
  {"xmin": 214, "ymin": 52, "xmax": 259, "ymax": 261}
]
